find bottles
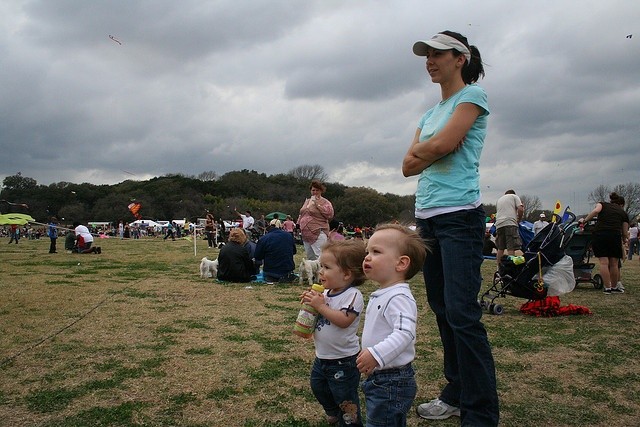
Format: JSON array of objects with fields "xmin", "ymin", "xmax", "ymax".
[{"xmin": 292, "ymin": 283, "xmax": 322, "ymax": 341}]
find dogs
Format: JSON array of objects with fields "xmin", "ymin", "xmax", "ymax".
[
  {"xmin": 297, "ymin": 255, "xmax": 321, "ymax": 287},
  {"xmin": 199, "ymin": 255, "xmax": 220, "ymax": 281}
]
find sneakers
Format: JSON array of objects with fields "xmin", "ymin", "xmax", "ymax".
[
  {"xmin": 617, "ymin": 281, "xmax": 625, "ymax": 289},
  {"xmin": 93, "ymin": 245, "xmax": 98, "ymax": 253},
  {"xmin": 612, "ymin": 284, "xmax": 624, "ymax": 293},
  {"xmin": 417, "ymin": 396, "xmax": 462, "ymax": 418},
  {"xmin": 97, "ymin": 246, "xmax": 101, "ymax": 254},
  {"xmin": 603, "ymin": 287, "xmax": 612, "ymax": 294}
]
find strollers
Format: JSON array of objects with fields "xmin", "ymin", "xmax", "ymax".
[
  {"xmin": 477, "ymin": 211, "xmax": 576, "ymax": 315},
  {"xmin": 559, "ymin": 215, "xmax": 604, "ymax": 290}
]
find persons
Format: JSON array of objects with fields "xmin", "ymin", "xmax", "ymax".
[
  {"xmin": 578, "ymin": 192, "xmax": 630, "ymax": 295},
  {"xmin": 402, "ymin": 30, "xmax": 500, "ymax": 427},
  {"xmin": 118, "ymin": 218, "xmax": 157, "ymax": 239},
  {"xmin": 65, "ymin": 226, "xmax": 79, "ymax": 250},
  {"xmin": 356, "ymin": 224, "xmax": 433, "ymax": 427},
  {"xmin": 254, "ymin": 225, "xmax": 297, "ymax": 282},
  {"xmin": 205, "ymin": 214, "xmax": 218, "ymax": 248},
  {"xmin": 74, "ymin": 222, "xmax": 101, "ymax": 254},
  {"xmin": 299, "ymin": 240, "xmax": 369, "ymax": 427},
  {"xmin": 160, "ymin": 220, "xmax": 192, "ymax": 241},
  {"xmin": 329, "ymin": 220, "xmax": 374, "ymax": 240},
  {"xmin": 628, "ymin": 223, "xmax": 640, "ymax": 260},
  {"xmin": 296, "ymin": 180, "xmax": 334, "ymax": 260},
  {"xmin": 496, "ymin": 190, "xmax": 524, "ymax": 278},
  {"xmin": 48, "ymin": 217, "xmax": 59, "ymax": 253},
  {"xmin": 218, "ymin": 210, "xmax": 301, "ymax": 238},
  {"xmin": 217, "ymin": 228, "xmax": 254, "ymax": 283},
  {"xmin": 240, "ymin": 228, "xmax": 263, "ymax": 275},
  {"xmin": 0, "ymin": 224, "xmax": 48, "ymax": 245},
  {"xmin": 532, "ymin": 213, "xmax": 549, "ymax": 237}
]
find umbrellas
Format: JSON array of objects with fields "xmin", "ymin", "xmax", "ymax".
[{"xmin": 263, "ymin": 210, "xmax": 290, "ymax": 222}]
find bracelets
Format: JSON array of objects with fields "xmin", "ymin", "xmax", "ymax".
[{"xmin": 316, "ymin": 204, "xmax": 321, "ymax": 208}]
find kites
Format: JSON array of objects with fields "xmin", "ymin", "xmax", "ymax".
[
  {"xmin": 0, "ymin": 213, "xmax": 193, "ymax": 244},
  {"xmin": 128, "ymin": 203, "xmax": 144, "ymax": 220}
]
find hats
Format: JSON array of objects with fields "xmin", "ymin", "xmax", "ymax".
[
  {"xmin": 540, "ymin": 213, "xmax": 545, "ymax": 217},
  {"xmin": 412, "ymin": 33, "xmax": 471, "ymax": 65}
]
